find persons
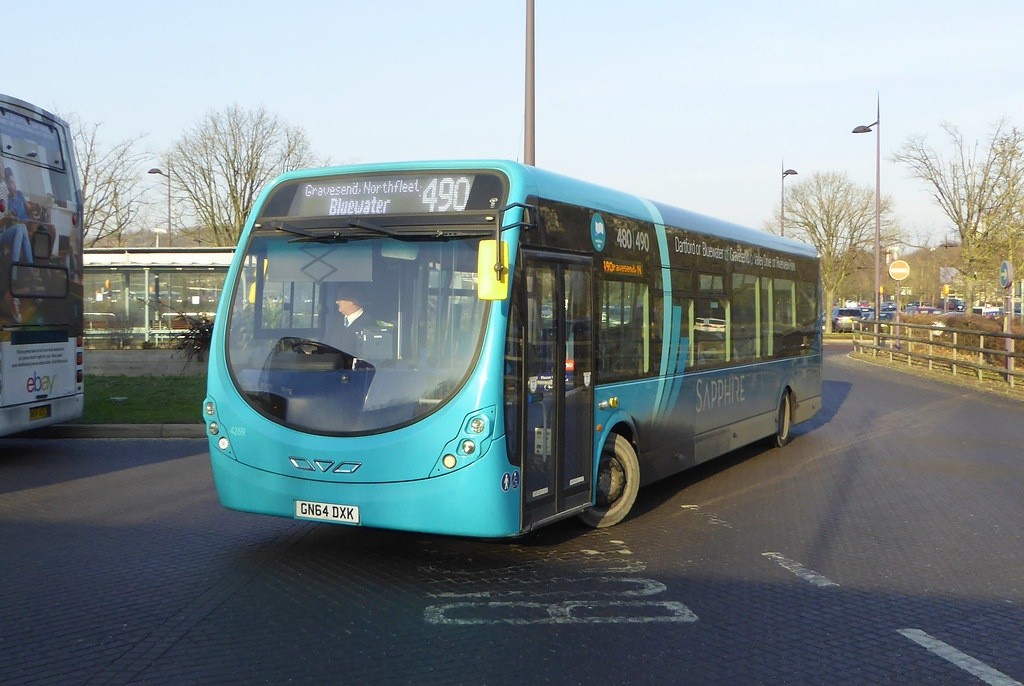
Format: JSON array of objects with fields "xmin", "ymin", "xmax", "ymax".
[
  {"xmin": 325, "ymin": 282, "xmax": 379, "ymax": 355},
  {"xmin": 95, "ymin": 290, "xmax": 103, "ymax": 301},
  {"xmin": 0, "ymin": 155, "xmax": 42, "ymax": 322}
]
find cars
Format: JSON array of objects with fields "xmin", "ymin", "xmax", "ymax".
[
  {"xmin": 820, "ymin": 297, "xmax": 1021, "ymax": 330},
  {"xmin": 830, "ymin": 307, "xmax": 866, "ymax": 333}
]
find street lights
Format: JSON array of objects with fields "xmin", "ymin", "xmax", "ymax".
[
  {"xmin": 851, "ymin": 91, "xmax": 881, "ymax": 348},
  {"xmin": 147, "ymin": 155, "xmax": 173, "ymax": 330},
  {"xmin": 779, "ymin": 158, "xmax": 798, "ymax": 236},
  {"xmin": 939, "ymin": 241, "xmax": 958, "ymax": 313}
]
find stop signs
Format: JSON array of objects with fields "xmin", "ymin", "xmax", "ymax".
[{"xmin": 889, "ymin": 260, "xmax": 910, "ymax": 281}]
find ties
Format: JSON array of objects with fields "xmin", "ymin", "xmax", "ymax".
[{"xmin": 344, "ymin": 317, "xmax": 349, "ymax": 327}]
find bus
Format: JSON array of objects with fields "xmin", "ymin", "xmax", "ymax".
[
  {"xmin": 201, "ymin": 158, "xmax": 822, "ymax": 539},
  {"xmin": 0, "ymin": 92, "xmax": 86, "ymax": 438}
]
